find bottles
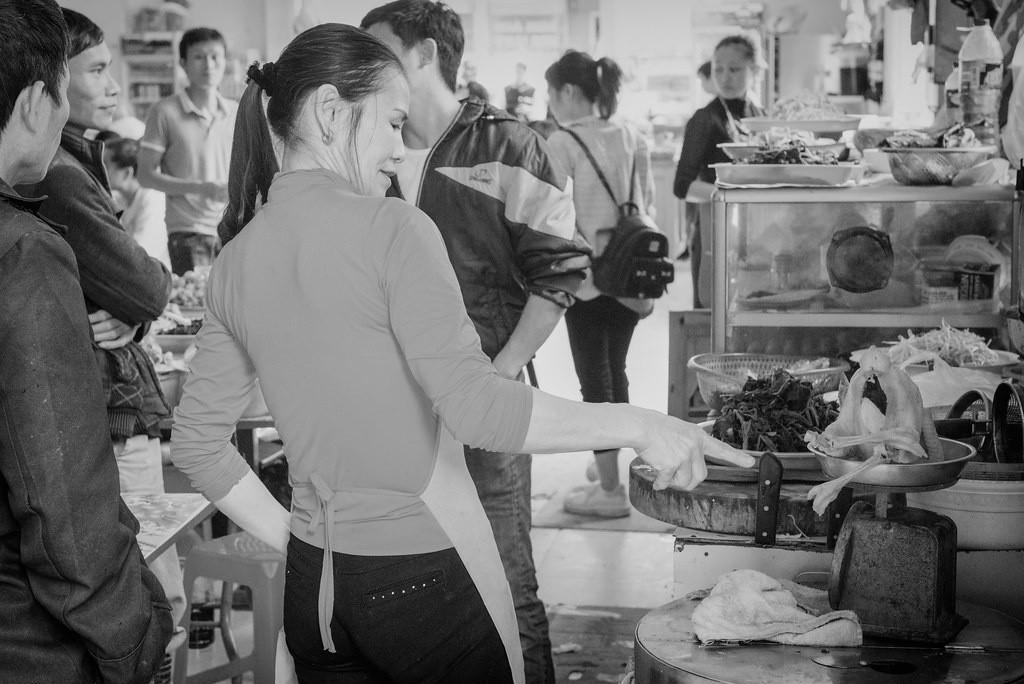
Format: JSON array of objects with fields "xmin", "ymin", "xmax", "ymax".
[
  {"xmin": 945, "ymin": 60, "xmax": 965, "ymax": 124},
  {"xmin": 916, "ymin": 256, "xmax": 958, "ymax": 305},
  {"xmin": 770, "ymin": 254, "xmax": 796, "ymax": 291},
  {"xmin": 955, "ymin": 18, "xmax": 1001, "ymax": 157}
]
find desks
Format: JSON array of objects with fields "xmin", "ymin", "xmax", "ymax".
[{"xmin": 120, "ymin": 492, "xmax": 218, "ymax": 563}]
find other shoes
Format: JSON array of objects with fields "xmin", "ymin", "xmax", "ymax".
[
  {"xmin": 189, "ymin": 603, "xmax": 215, "ymax": 648},
  {"xmin": 230, "ymin": 584, "xmax": 253, "ymax": 610}
]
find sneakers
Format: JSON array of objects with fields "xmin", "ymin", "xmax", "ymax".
[
  {"xmin": 562, "ymin": 481, "xmax": 632, "ymax": 517},
  {"xmin": 586, "ymin": 461, "xmax": 600, "ymax": 480}
]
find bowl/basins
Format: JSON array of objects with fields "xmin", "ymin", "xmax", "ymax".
[{"xmin": 1005, "ymin": 309, "xmax": 1024, "ymax": 356}]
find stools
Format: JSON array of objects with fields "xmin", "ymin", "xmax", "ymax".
[{"xmin": 173, "ymin": 532, "xmax": 297, "ymax": 684}]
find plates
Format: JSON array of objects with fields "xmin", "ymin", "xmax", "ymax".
[
  {"xmin": 693, "ymin": 420, "xmax": 822, "ymax": 470},
  {"xmin": 154, "ymin": 334, "xmax": 198, "ymax": 351}
]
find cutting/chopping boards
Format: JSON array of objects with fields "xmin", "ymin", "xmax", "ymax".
[{"xmin": 630, "ymin": 456, "xmax": 879, "ymax": 535}]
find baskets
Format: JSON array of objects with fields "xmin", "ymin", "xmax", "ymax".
[
  {"xmin": 687, "ymin": 351, "xmax": 851, "ymax": 411},
  {"xmin": 884, "ymin": 145, "xmax": 992, "ymax": 185}
]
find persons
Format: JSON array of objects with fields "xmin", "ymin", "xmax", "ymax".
[
  {"xmin": 95, "ymin": 127, "xmax": 174, "ymax": 270},
  {"xmin": 18, "ymin": 7, "xmax": 186, "ymax": 684},
  {"xmin": 459, "ymin": 60, "xmax": 489, "ymax": 102},
  {"xmin": 172, "ymin": 23, "xmax": 755, "ymax": 684},
  {"xmin": 698, "ymin": 62, "xmax": 718, "ymax": 96},
  {"xmin": 356, "ymin": 0, "xmax": 589, "ymax": 684},
  {"xmin": 503, "ymin": 62, "xmax": 534, "ymax": 126},
  {"xmin": 136, "ymin": 26, "xmax": 238, "ymax": 280},
  {"xmin": 674, "ymin": 35, "xmax": 765, "ymax": 310},
  {"xmin": 540, "ymin": 50, "xmax": 656, "ymax": 515},
  {"xmin": 0, "ymin": 0, "xmax": 176, "ymax": 684}
]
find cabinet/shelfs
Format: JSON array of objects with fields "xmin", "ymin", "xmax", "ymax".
[{"xmin": 119, "ymin": 34, "xmax": 190, "ymax": 125}]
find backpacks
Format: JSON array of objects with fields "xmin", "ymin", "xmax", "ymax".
[{"xmin": 559, "ymin": 126, "xmax": 674, "ymax": 299}]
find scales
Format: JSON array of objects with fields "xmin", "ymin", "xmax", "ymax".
[{"xmin": 806, "ymin": 435, "xmax": 977, "ymax": 646}]
